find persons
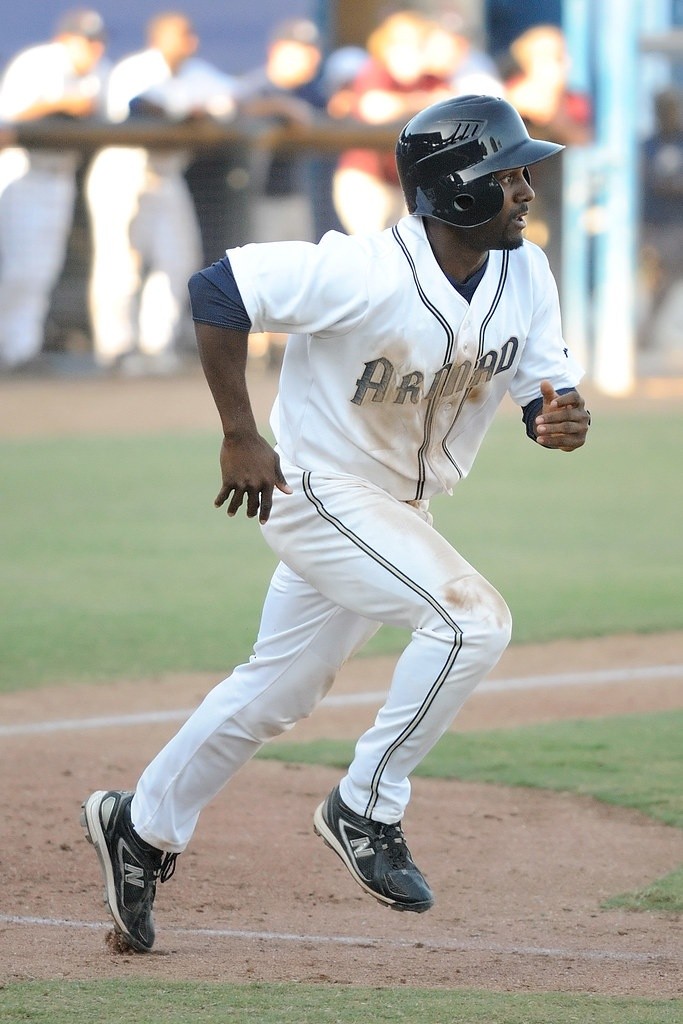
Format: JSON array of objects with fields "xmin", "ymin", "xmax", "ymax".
[
  {"xmin": 0, "ymin": 8, "xmax": 592, "ymax": 372},
  {"xmin": 84, "ymin": 95, "xmax": 590, "ymax": 954}
]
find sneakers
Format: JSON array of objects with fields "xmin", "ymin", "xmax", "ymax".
[
  {"xmin": 313, "ymin": 784, "xmax": 435, "ymax": 913},
  {"xmin": 79, "ymin": 789, "xmax": 181, "ymax": 953}
]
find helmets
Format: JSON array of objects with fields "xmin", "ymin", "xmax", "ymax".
[{"xmin": 393, "ymin": 95, "xmax": 566, "ymax": 228}]
